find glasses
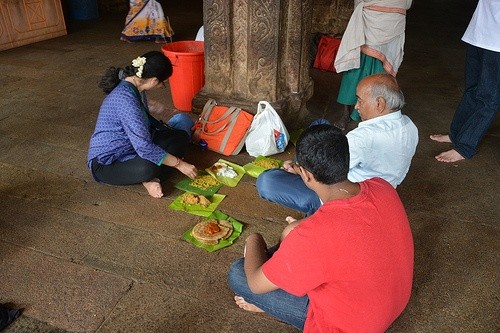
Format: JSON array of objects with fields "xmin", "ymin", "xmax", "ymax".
[{"xmin": 159, "ymin": 79, "xmax": 167, "ymax": 89}]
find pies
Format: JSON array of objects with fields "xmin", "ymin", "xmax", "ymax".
[{"xmin": 192, "ymin": 219, "xmax": 234, "ymax": 244}]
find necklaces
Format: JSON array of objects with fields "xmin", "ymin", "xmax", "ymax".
[
  {"xmin": 132, "ymin": 76, "xmax": 140, "ymax": 92},
  {"xmin": 322, "ymin": 180, "xmax": 350, "ymax": 204}
]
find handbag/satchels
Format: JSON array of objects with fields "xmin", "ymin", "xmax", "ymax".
[
  {"xmin": 314, "ymin": 34, "xmax": 343, "ymax": 71},
  {"xmin": 193, "ymin": 97, "xmax": 255, "ymax": 157}
]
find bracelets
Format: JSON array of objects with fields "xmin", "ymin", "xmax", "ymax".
[{"xmin": 174, "ymin": 157, "xmax": 181, "ymax": 167}]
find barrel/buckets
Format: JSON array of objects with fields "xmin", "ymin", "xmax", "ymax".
[{"xmin": 160, "ymin": 40, "xmax": 205, "ymax": 111}]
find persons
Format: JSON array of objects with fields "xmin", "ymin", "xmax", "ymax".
[
  {"xmin": 87, "ymin": 50, "xmax": 199, "ymax": 198},
  {"xmin": 430, "ymin": 0, "xmax": 500, "ymax": 162},
  {"xmin": 255, "ymin": 73, "xmax": 418, "ymax": 218},
  {"xmin": 227, "ymin": 125, "xmax": 414, "ymax": 333},
  {"xmin": 120, "ymin": 0, "xmax": 175, "ymax": 45},
  {"xmin": 334, "ymin": 0, "xmax": 412, "ymax": 130}
]
